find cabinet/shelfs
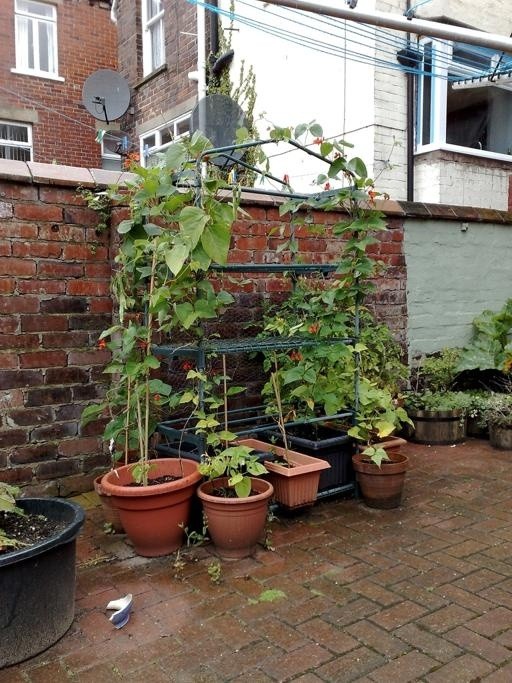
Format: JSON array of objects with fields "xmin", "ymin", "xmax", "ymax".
[{"xmin": 145, "ymin": 128, "xmax": 360, "ymax": 462}]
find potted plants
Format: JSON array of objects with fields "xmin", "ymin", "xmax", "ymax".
[
  {"xmin": 327, "ymin": 420, "xmax": 410, "ymax": 453},
  {"xmin": 351, "ymin": 331, "xmax": 414, "ymax": 512},
  {"xmin": 99, "ymin": 318, "xmax": 205, "ymax": 557},
  {"xmin": 195, "ymin": 427, "xmax": 275, "ymax": 560},
  {"xmin": 266, "ymin": 348, "xmax": 354, "ymax": 493},
  {"xmin": 229, "ymin": 364, "xmax": 332, "ymax": 511},
  {"xmin": 405, "ymin": 383, "xmax": 512, "ymax": 448},
  {"xmin": 80, "ymin": 356, "xmax": 156, "ymax": 530},
  {"xmin": 1, "ymin": 476, "xmax": 86, "ymax": 674}
]
